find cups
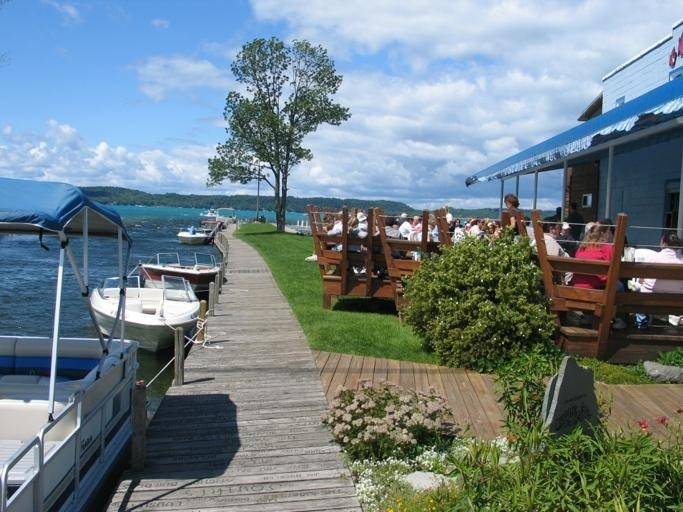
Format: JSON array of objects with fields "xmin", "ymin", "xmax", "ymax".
[{"xmin": 623, "ymin": 246, "xmax": 635, "ymax": 262}]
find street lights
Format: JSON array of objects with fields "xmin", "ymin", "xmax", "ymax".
[{"xmin": 252, "ymin": 157, "xmax": 260, "ymax": 221}]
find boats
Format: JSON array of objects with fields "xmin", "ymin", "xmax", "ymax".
[
  {"xmin": 137, "ymin": 251, "xmax": 221, "ymax": 296},
  {"xmin": 199, "ymin": 218, "xmax": 223, "ymax": 236},
  {"xmin": 177, "ymin": 226, "xmax": 214, "ymax": 246},
  {"xmin": 206, "ymin": 208, "xmax": 216, "ymax": 217},
  {"xmin": 0, "ymin": 173, "xmax": 145, "ymax": 512},
  {"xmin": 215, "ymin": 207, "xmax": 236, "ymax": 229},
  {"xmin": 89, "ymin": 269, "xmax": 201, "ymax": 356}
]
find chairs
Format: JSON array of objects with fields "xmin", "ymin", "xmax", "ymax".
[{"xmin": 300, "ymin": 205, "xmax": 682, "ymax": 368}]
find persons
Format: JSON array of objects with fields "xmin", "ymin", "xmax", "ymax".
[{"xmin": 321, "ymin": 194, "xmax": 682, "ymax": 330}]
